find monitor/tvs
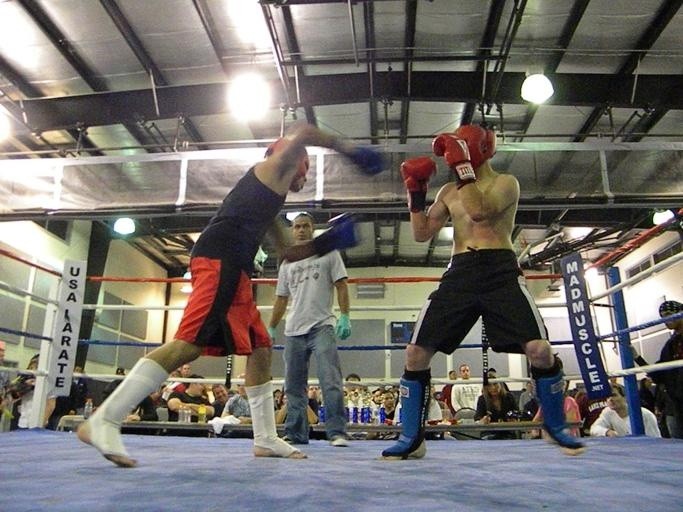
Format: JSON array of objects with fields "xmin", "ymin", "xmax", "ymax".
[{"xmin": 391, "ymin": 321, "xmax": 416, "ymax": 343}]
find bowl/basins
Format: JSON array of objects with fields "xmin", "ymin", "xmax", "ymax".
[{"xmin": 425, "ymin": 420, "xmax": 440, "ymax": 425}]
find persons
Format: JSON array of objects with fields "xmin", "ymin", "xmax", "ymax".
[
  {"xmin": 0, "ymin": 340, "xmax": 681, "ymax": 440},
  {"xmin": 628, "ymin": 301, "xmax": 682, "ymax": 438},
  {"xmin": 77, "ymin": 122, "xmax": 391, "ymax": 466},
  {"xmin": 382, "ymin": 124, "xmax": 586, "ymax": 458},
  {"xmin": 268, "ymin": 214, "xmax": 352, "ymax": 446}
]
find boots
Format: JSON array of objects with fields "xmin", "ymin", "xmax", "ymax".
[
  {"xmin": 530, "ymin": 355, "xmax": 585, "ymax": 455},
  {"xmin": 379, "ymin": 366, "xmax": 432, "ymax": 461}
]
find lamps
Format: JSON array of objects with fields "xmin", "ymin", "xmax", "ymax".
[{"xmin": 520, "ymin": 65, "xmax": 553, "ymax": 106}]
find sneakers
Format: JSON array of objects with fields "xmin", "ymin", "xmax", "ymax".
[
  {"xmin": 329, "ymin": 435, "xmax": 349, "ymax": 447},
  {"xmin": 282, "ymin": 435, "xmax": 295, "ymax": 444}
]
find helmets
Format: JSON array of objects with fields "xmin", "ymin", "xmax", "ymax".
[
  {"xmin": 456, "ymin": 125, "xmax": 496, "ymax": 169},
  {"xmin": 659, "ymin": 300, "xmax": 683, "ymax": 316},
  {"xmin": 267, "ymin": 137, "xmax": 310, "ymax": 179}
]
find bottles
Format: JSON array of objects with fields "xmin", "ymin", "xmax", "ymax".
[
  {"xmin": 177, "ymin": 406, "xmax": 192, "ymax": 423},
  {"xmin": 197, "ymin": 403, "xmax": 207, "ymax": 425},
  {"xmin": 83, "ymin": 398, "xmax": 93, "ymax": 420},
  {"xmin": 316, "ymin": 403, "xmax": 386, "ymax": 425}
]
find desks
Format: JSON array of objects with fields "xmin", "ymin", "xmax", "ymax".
[
  {"xmin": 61, "ymin": 416, "xmax": 312, "ymax": 438},
  {"xmin": 313, "ymin": 422, "xmax": 584, "ymax": 438}
]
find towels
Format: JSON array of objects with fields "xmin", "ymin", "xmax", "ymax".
[{"xmin": 208, "ymin": 414, "xmax": 241, "ymax": 434}]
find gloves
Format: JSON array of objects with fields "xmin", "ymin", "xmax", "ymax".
[
  {"xmin": 351, "ymin": 147, "xmax": 385, "ymax": 175},
  {"xmin": 316, "ymin": 211, "xmax": 357, "ymax": 255},
  {"xmin": 400, "ymin": 156, "xmax": 437, "ymax": 212},
  {"xmin": 266, "ymin": 327, "xmax": 276, "ymax": 341},
  {"xmin": 431, "ymin": 132, "xmax": 477, "ymax": 189},
  {"xmin": 334, "ymin": 314, "xmax": 351, "ymax": 339}
]
